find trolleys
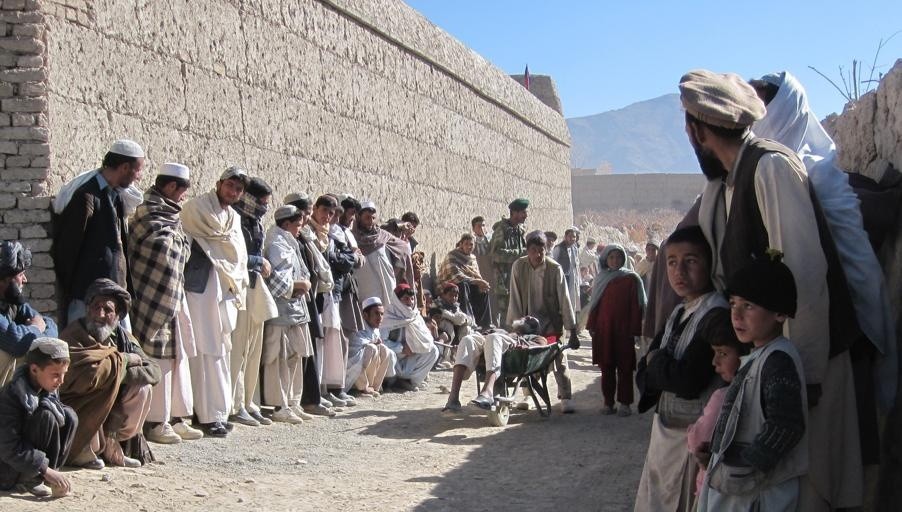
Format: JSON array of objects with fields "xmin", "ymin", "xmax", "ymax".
[{"xmin": 474, "ymin": 335, "xmax": 575, "ymax": 427}]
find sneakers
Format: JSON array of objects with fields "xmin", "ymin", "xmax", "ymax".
[
  {"xmin": 204, "ymin": 422, "xmax": 228, "ymax": 437},
  {"xmin": 249, "ymin": 410, "xmax": 272, "ymax": 425},
  {"xmin": 145, "ymin": 422, "xmax": 181, "ymax": 443},
  {"xmin": 229, "ymin": 408, "xmax": 261, "ymax": 426},
  {"xmin": 600, "ymin": 408, "xmax": 616, "ymax": 415},
  {"xmin": 325, "ymin": 393, "xmax": 346, "ymax": 407},
  {"xmin": 320, "ymin": 396, "xmax": 333, "ymax": 407},
  {"xmin": 618, "ymin": 405, "xmax": 631, "ymax": 417},
  {"xmin": 30, "ymin": 484, "xmax": 53, "ymax": 495},
  {"xmin": 560, "ymin": 399, "xmax": 575, "ymax": 414},
  {"xmin": 171, "ymin": 422, "xmax": 204, "ymax": 439},
  {"xmin": 516, "ymin": 395, "xmax": 535, "ymax": 410},
  {"xmin": 304, "ymin": 404, "xmax": 336, "ymax": 416},
  {"xmin": 272, "ymin": 408, "xmax": 303, "ymax": 424},
  {"xmin": 335, "ymin": 393, "xmax": 356, "ymax": 406},
  {"xmin": 116, "ymin": 456, "xmax": 141, "ymax": 467},
  {"xmin": 83, "ymin": 459, "xmax": 104, "ymax": 469},
  {"xmin": 290, "ymin": 406, "xmax": 313, "ymax": 420}
]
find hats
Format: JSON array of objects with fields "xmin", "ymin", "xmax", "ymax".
[
  {"xmin": 444, "ymin": 283, "xmax": 458, "ymax": 289},
  {"xmin": 630, "ymin": 246, "xmax": 639, "ymax": 251},
  {"xmin": 283, "ymin": 191, "xmax": 308, "ymax": 205},
  {"xmin": 597, "ymin": 243, "xmax": 606, "ymax": 251},
  {"xmin": 508, "ymin": 198, "xmax": 529, "ymax": 211},
  {"xmin": 26, "ymin": 337, "xmax": 71, "ymax": 360},
  {"xmin": 361, "ymin": 297, "xmax": 382, "ymax": 310},
  {"xmin": 274, "ymin": 205, "xmax": 297, "ymax": 220},
  {"xmin": 109, "ymin": 139, "xmax": 144, "ymax": 157},
  {"xmin": 525, "ymin": 229, "xmax": 547, "ymax": 243},
  {"xmin": 158, "ymin": 163, "xmax": 189, "ymax": 180},
  {"xmin": 678, "ymin": 69, "xmax": 767, "ymax": 129},
  {"xmin": 394, "ymin": 284, "xmax": 411, "ymax": 294},
  {"xmin": 360, "ymin": 202, "xmax": 375, "ymax": 208},
  {"xmin": 220, "ymin": 165, "xmax": 248, "ymax": 181},
  {"xmin": 647, "ymin": 239, "xmax": 660, "ymax": 250},
  {"xmin": 720, "ymin": 248, "xmax": 798, "ymax": 320},
  {"xmin": 587, "ymin": 237, "xmax": 596, "ymax": 243}
]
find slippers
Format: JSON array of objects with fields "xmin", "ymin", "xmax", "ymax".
[
  {"xmin": 442, "ymin": 403, "xmax": 463, "ymax": 412},
  {"xmin": 467, "ymin": 395, "xmax": 496, "ymax": 412}
]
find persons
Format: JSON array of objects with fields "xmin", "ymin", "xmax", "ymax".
[
  {"xmin": 595, "ymin": 243, "xmax": 606, "ymax": 276},
  {"xmin": 0, "ymin": 241, "xmax": 59, "ymax": 393},
  {"xmin": 379, "ymin": 218, "xmax": 416, "ymax": 242},
  {"xmin": 634, "ymin": 224, "xmax": 756, "ymax": 512},
  {"xmin": 53, "ymin": 140, "xmax": 157, "ymax": 468},
  {"xmin": 336, "ymin": 193, "xmax": 368, "ymax": 333},
  {"xmin": 423, "ymin": 307, "xmax": 452, "ymax": 373},
  {"xmin": 124, "ymin": 161, "xmax": 206, "ymax": 445},
  {"xmin": 623, "ymin": 245, "xmax": 643, "ymax": 271},
  {"xmin": 570, "ymin": 226, "xmax": 581, "ymax": 248},
  {"xmin": 263, "ymin": 203, "xmax": 314, "ymax": 422},
  {"xmin": 321, "ymin": 194, "xmax": 357, "ymax": 408},
  {"xmin": 684, "ymin": 310, "xmax": 754, "ymax": 499},
  {"xmin": 440, "ymin": 311, "xmax": 547, "ymax": 416},
  {"xmin": 585, "ymin": 245, "xmax": 648, "ymax": 417},
  {"xmin": 401, "ymin": 212, "xmax": 420, "ymax": 227},
  {"xmin": 431, "ymin": 233, "xmax": 497, "ymax": 331},
  {"xmin": 635, "ymin": 239, "xmax": 660, "ymax": 347},
  {"xmin": 379, "ymin": 284, "xmax": 440, "ymax": 392},
  {"xmin": 1, "ymin": 336, "xmax": 80, "ymax": 501},
  {"xmin": 351, "ymin": 202, "xmax": 415, "ymax": 306},
  {"xmin": 679, "ymin": 68, "xmax": 865, "ymax": 512},
  {"xmin": 434, "ymin": 283, "xmax": 473, "ymax": 366},
  {"xmin": 471, "ymin": 216, "xmax": 492, "ymax": 273},
  {"xmin": 578, "ymin": 266, "xmax": 594, "ymax": 309},
  {"xmin": 582, "ymin": 237, "xmax": 597, "ymax": 279},
  {"xmin": 693, "ymin": 252, "xmax": 811, "ymax": 510},
  {"xmin": 232, "ymin": 175, "xmax": 275, "ymax": 427},
  {"xmin": 552, "ymin": 228, "xmax": 583, "ymax": 339},
  {"xmin": 746, "ymin": 71, "xmax": 902, "ymax": 510},
  {"xmin": 284, "ymin": 193, "xmax": 335, "ymax": 416},
  {"xmin": 485, "ymin": 198, "xmax": 536, "ymax": 334},
  {"xmin": 58, "ymin": 275, "xmax": 164, "ymax": 472},
  {"xmin": 182, "ymin": 167, "xmax": 252, "ymax": 439},
  {"xmin": 544, "ymin": 231, "xmax": 557, "ymax": 258},
  {"xmin": 505, "ymin": 228, "xmax": 582, "ymax": 415},
  {"xmin": 346, "ymin": 296, "xmax": 398, "ymax": 397}
]
